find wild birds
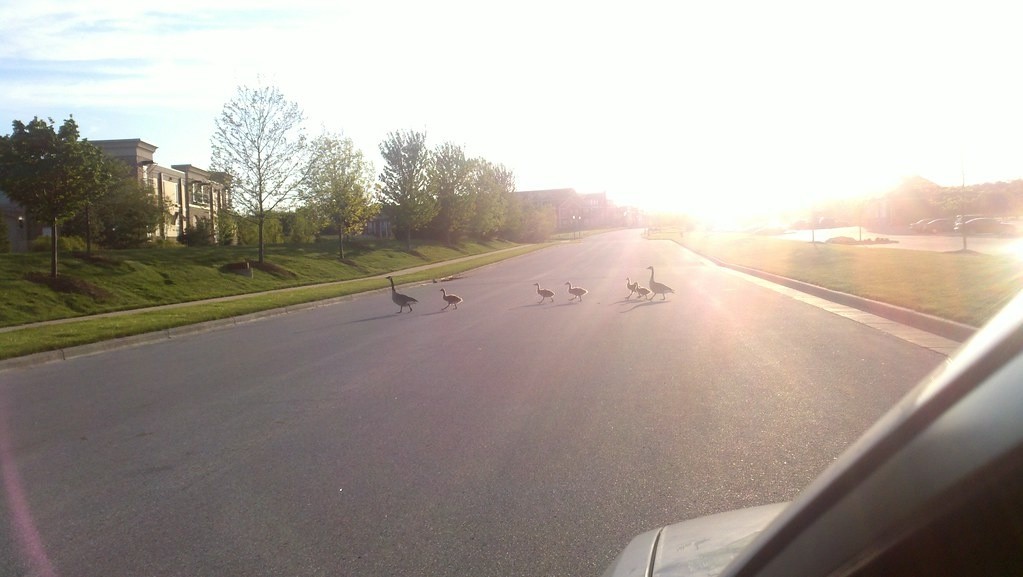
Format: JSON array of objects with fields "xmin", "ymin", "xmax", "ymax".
[
  {"xmin": 625, "ymin": 277, "xmax": 636, "ymax": 299},
  {"xmin": 439, "ymin": 288, "xmax": 463, "ymax": 310},
  {"xmin": 386, "ymin": 276, "xmax": 419, "ymax": 313},
  {"xmin": 646, "ymin": 265, "xmax": 675, "ymax": 301},
  {"xmin": 533, "ymin": 283, "xmax": 555, "ymax": 304},
  {"xmin": 633, "ymin": 282, "xmax": 650, "ymax": 299},
  {"xmin": 565, "ymin": 282, "xmax": 588, "ymax": 302}
]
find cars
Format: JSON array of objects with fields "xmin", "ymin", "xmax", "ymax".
[{"xmin": 912, "ymin": 214, "xmax": 1016, "ymax": 236}]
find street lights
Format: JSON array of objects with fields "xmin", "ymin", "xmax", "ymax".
[
  {"xmin": 578, "ymin": 214, "xmax": 581, "ymax": 239},
  {"xmin": 571, "ymin": 215, "xmax": 576, "ymax": 240}
]
